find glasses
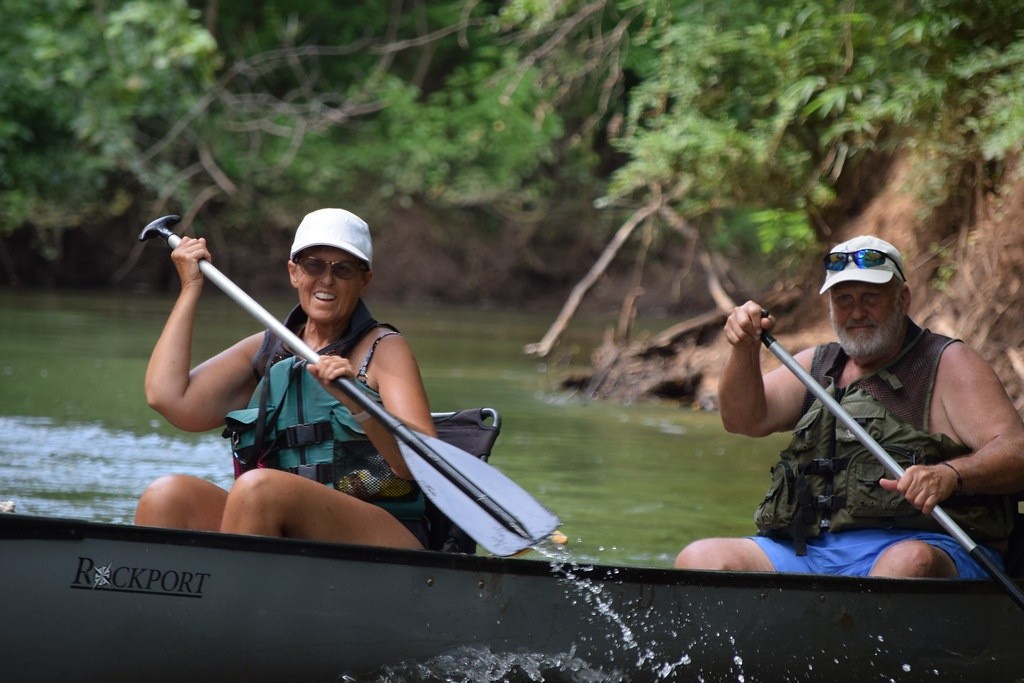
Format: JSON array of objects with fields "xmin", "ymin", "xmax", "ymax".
[
  {"xmin": 823, "ymin": 248, "xmax": 907, "ymax": 282},
  {"xmin": 293, "ymin": 254, "xmax": 360, "ymax": 280}
]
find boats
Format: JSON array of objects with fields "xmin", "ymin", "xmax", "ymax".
[{"xmin": 0, "ymin": 512, "xmax": 1024, "ymax": 683}]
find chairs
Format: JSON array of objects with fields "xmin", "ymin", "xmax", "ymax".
[{"xmin": 424, "ymin": 404, "xmax": 504, "ymax": 553}]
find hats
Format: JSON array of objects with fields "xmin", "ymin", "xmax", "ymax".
[
  {"xmin": 290, "ymin": 208, "xmax": 373, "ymax": 270},
  {"xmin": 819, "ymin": 235, "xmax": 905, "ymax": 296}
]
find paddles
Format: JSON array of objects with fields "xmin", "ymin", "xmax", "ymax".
[
  {"xmin": 138, "ymin": 213, "xmax": 559, "ymax": 558},
  {"xmin": 754, "ymin": 309, "xmax": 1022, "ymax": 606}
]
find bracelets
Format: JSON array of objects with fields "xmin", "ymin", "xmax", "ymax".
[
  {"xmin": 351, "ymin": 412, "xmax": 370, "ymax": 423},
  {"xmin": 941, "ymin": 462, "xmax": 962, "ymax": 494}
]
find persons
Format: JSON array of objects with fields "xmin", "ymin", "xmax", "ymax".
[
  {"xmin": 673, "ymin": 236, "xmax": 1024, "ymax": 578},
  {"xmin": 136, "ymin": 209, "xmax": 441, "ymax": 550}
]
